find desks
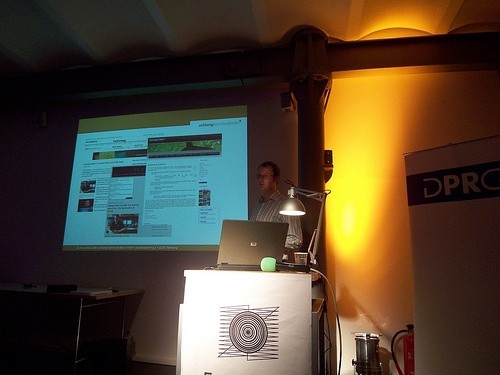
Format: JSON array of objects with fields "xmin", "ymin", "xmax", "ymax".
[{"xmin": 0, "ymin": 282, "xmax": 140, "ymax": 375}]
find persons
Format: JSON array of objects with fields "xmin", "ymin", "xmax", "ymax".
[
  {"xmin": 107, "ymin": 214, "xmax": 126, "ymax": 233},
  {"xmin": 79, "ymin": 200, "xmax": 93, "ymax": 211},
  {"xmin": 250, "ymin": 161, "xmax": 303, "ymax": 264},
  {"xmin": 80, "ymin": 181, "xmax": 92, "ymax": 193}
]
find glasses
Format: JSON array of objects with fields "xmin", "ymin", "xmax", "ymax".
[{"xmin": 255, "ymin": 173, "xmax": 273, "ymax": 178}]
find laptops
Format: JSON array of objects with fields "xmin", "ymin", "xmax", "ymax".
[{"xmin": 218, "ymin": 217, "xmax": 289, "ymax": 265}]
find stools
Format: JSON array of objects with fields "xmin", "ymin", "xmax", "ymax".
[{"xmin": 79, "ymin": 336, "xmax": 136, "ymax": 375}]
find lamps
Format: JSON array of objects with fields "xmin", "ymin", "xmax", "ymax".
[{"xmin": 279, "ymin": 186, "xmax": 326, "ymax": 267}]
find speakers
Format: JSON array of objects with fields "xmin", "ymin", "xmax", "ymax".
[{"xmin": 281, "ymin": 91, "xmax": 293, "ymax": 114}]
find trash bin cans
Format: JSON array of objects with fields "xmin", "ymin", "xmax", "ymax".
[{"xmin": 391, "ymin": 324, "xmax": 415, "ymax": 375}]
